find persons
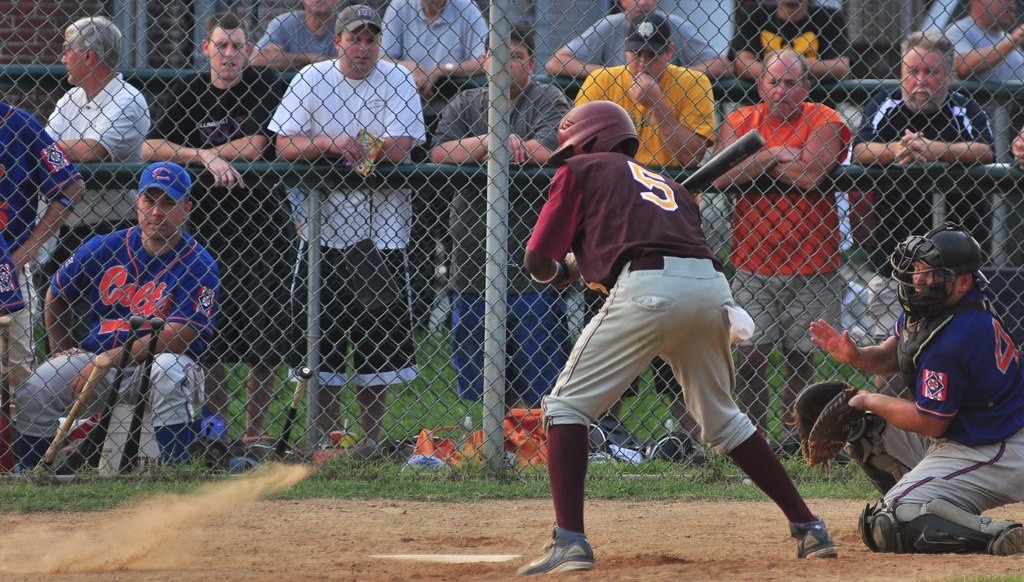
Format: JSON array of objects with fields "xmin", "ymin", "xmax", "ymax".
[
  {"xmin": 14, "ymin": 162, "xmax": 220, "ymax": 474},
  {"xmin": 810, "ymin": 222, "xmax": 1024, "ymax": 557},
  {"xmin": 545, "ymin": 0, "xmax": 1024, "ymax": 459},
  {"xmin": 0, "ymin": 100, "xmax": 85, "ymax": 471},
  {"xmin": 514, "ymin": 100, "xmax": 838, "ymax": 576},
  {"xmin": 45, "ymin": 0, "xmax": 573, "ymax": 456}
]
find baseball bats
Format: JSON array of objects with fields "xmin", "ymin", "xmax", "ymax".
[
  {"xmin": 273, "ymin": 365, "xmax": 312, "ymax": 462},
  {"xmin": 38, "ymin": 354, "xmax": 108, "ymax": 473},
  {"xmin": 679, "ymin": 126, "xmax": 767, "ymax": 194}
]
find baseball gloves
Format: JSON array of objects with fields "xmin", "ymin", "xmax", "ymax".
[{"xmin": 784, "ymin": 379, "xmax": 869, "ymax": 477}]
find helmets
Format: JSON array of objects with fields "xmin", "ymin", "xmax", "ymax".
[
  {"xmin": 909, "ymin": 221, "xmax": 988, "ymax": 283},
  {"xmin": 547, "ymin": 100, "xmax": 638, "ymax": 168}
]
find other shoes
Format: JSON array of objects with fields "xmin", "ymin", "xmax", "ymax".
[{"xmin": 992, "ymin": 524, "xmax": 1024, "ymax": 556}]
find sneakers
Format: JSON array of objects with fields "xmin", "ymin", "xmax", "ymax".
[
  {"xmin": 790, "ymin": 516, "xmax": 838, "ymax": 560},
  {"xmin": 514, "ymin": 523, "xmax": 595, "ymax": 574}
]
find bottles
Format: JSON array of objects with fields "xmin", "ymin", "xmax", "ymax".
[{"xmin": 458, "ymin": 417, "xmax": 476, "ymax": 458}]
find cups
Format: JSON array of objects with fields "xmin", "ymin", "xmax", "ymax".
[{"xmin": 330, "ymin": 431, "xmax": 358, "ymax": 449}]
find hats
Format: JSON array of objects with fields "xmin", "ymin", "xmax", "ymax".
[
  {"xmin": 624, "ymin": 15, "xmax": 670, "ymax": 52},
  {"xmin": 334, "ymin": 4, "xmax": 383, "ymax": 35},
  {"xmin": 137, "ymin": 161, "xmax": 190, "ymax": 202}
]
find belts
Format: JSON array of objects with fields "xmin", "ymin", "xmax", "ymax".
[{"xmin": 629, "ymin": 257, "xmax": 724, "ymax": 272}]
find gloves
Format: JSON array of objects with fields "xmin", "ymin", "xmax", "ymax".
[{"xmin": 530, "ymin": 251, "xmax": 578, "ymax": 288}]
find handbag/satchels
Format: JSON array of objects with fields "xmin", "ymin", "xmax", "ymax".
[
  {"xmin": 503, "ymin": 408, "xmax": 550, "ymax": 468},
  {"xmin": 413, "ymin": 424, "xmax": 483, "ymax": 469},
  {"xmin": 648, "ymin": 430, "xmax": 693, "ymax": 463},
  {"xmin": 587, "ymin": 416, "xmax": 646, "ymax": 457}
]
